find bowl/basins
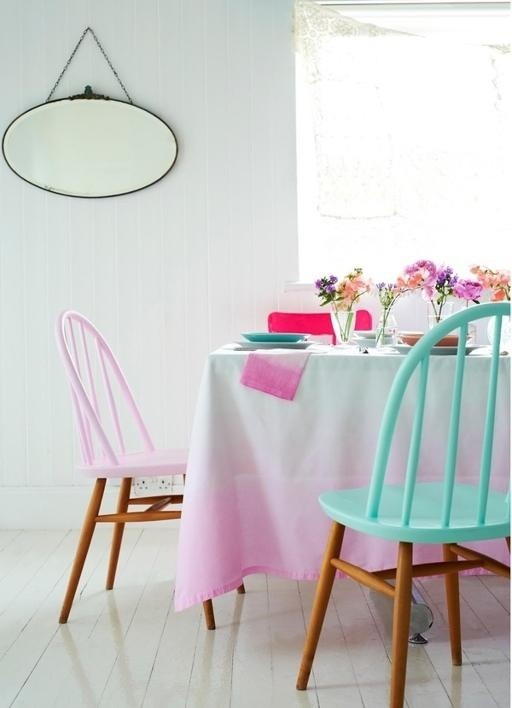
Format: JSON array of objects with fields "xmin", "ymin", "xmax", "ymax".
[{"xmin": 397, "ymin": 330, "xmax": 425, "ymax": 344}]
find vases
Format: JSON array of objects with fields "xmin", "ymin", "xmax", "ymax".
[{"xmin": 326, "ymin": 313, "xmax": 510, "ymax": 351}]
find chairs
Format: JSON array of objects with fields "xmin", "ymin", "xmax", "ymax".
[
  {"xmin": 294, "ymin": 302, "xmax": 510, "ymax": 708},
  {"xmin": 56, "ymin": 308, "xmax": 240, "ymax": 632}
]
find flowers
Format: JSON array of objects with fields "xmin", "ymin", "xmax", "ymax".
[{"xmin": 315, "ymin": 266, "xmax": 511, "ymax": 302}]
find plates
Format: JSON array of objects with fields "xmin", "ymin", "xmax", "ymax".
[
  {"xmin": 391, "ymin": 346, "xmax": 486, "ymax": 354},
  {"xmin": 398, "ymin": 336, "xmax": 471, "ymax": 345},
  {"xmin": 241, "ymin": 332, "xmax": 311, "ymax": 341},
  {"xmin": 235, "ymin": 341, "xmax": 314, "ymax": 349},
  {"xmin": 353, "ymin": 330, "xmax": 394, "ymax": 337},
  {"xmin": 353, "ymin": 339, "xmax": 398, "ymax": 347}
]
